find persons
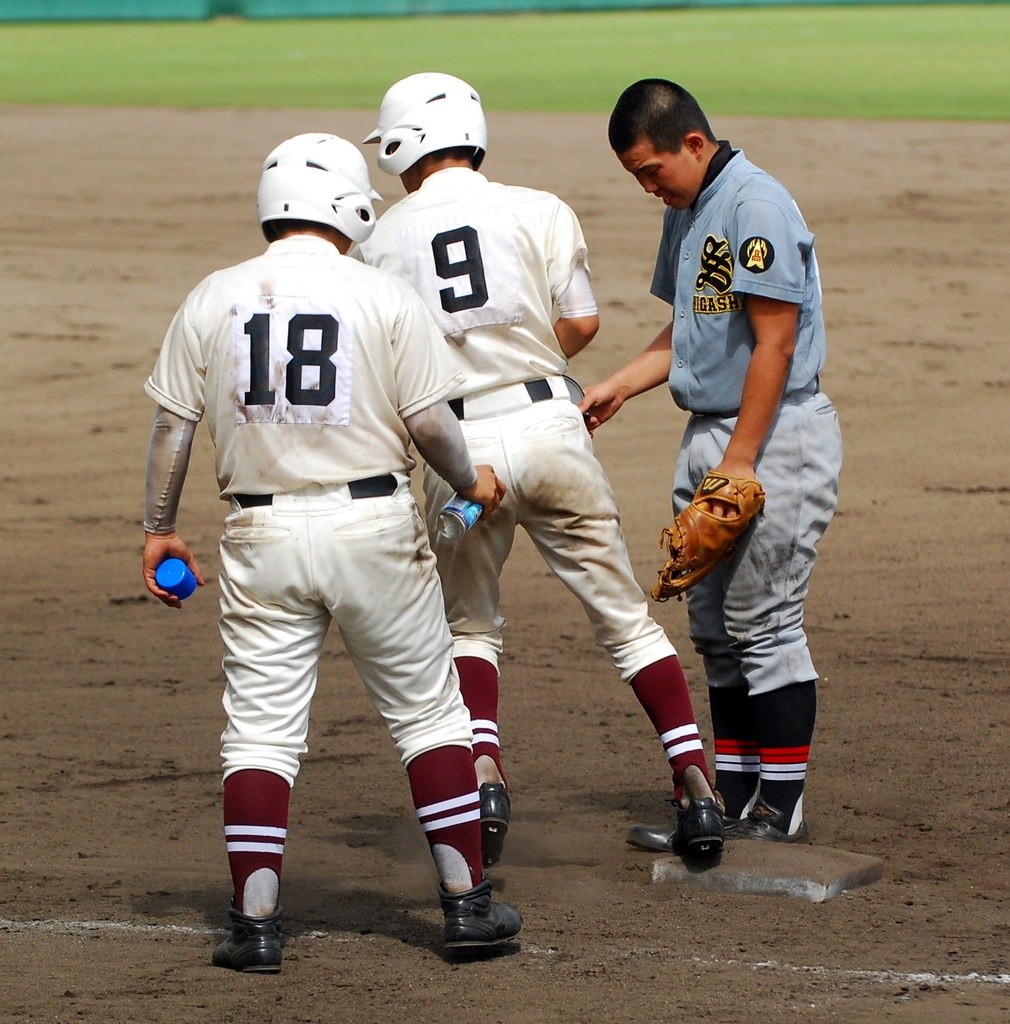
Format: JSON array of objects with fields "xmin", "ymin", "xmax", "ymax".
[
  {"xmin": 140, "ymin": 131, "xmax": 523, "ymax": 973},
  {"xmin": 347, "ymin": 72, "xmax": 725, "ymax": 866},
  {"xmin": 577, "ymin": 80, "xmax": 843, "ymax": 851}
]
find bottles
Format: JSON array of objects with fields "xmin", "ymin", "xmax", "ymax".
[{"xmin": 436, "ymin": 488, "xmax": 486, "ymax": 541}]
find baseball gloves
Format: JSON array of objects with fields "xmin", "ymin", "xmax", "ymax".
[{"xmin": 650, "ymin": 469, "xmax": 766, "ymax": 602}]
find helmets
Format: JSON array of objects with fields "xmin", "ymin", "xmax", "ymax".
[
  {"xmin": 361, "ymin": 72, "xmax": 486, "ymax": 176},
  {"xmin": 256, "ymin": 133, "xmax": 384, "ymax": 243}
]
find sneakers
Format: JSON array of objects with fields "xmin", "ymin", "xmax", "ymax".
[
  {"xmin": 479, "ymin": 782, "xmax": 511, "ymax": 870},
  {"xmin": 211, "ymin": 895, "xmax": 283, "ymax": 974},
  {"xmin": 724, "ymin": 797, "xmax": 811, "ymax": 844},
  {"xmin": 671, "ymin": 797, "xmax": 724, "ymax": 874},
  {"xmin": 436, "ymin": 874, "xmax": 524, "ymax": 950},
  {"xmin": 625, "ymin": 824, "xmax": 742, "ymax": 852}
]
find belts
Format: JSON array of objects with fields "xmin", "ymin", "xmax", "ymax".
[
  {"xmin": 447, "ymin": 376, "xmax": 552, "ymax": 420},
  {"xmin": 232, "ymin": 475, "xmax": 398, "ymax": 509}
]
